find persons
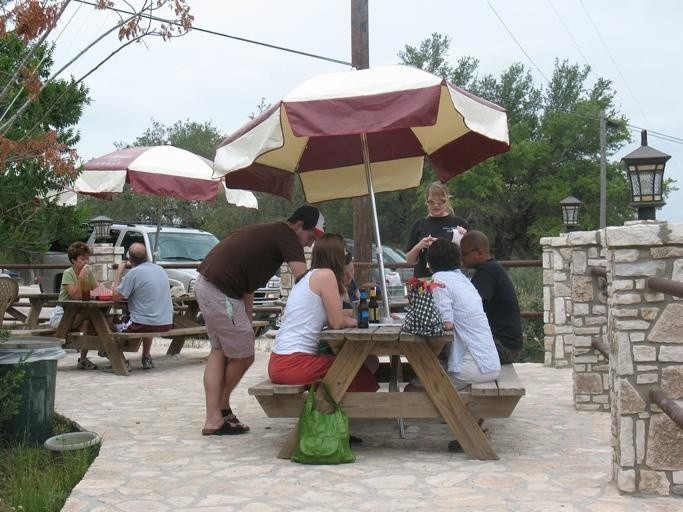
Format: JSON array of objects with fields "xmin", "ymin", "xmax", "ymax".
[
  {"xmin": 59, "ymin": 242, "xmax": 108, "ymax": 369},
  {"xmin": 319, "ymin": 250, "xmax": 362, "ymax": 354},
  {"xmin": 104, "ymin": 242, "xmax": 173, "ymax": 373},
  {"xmin": 460, "ymin": 230, "xmax": 523, "ymax": 364},
  {"xmin": 405, "ymin": 238, "xmax": 500, "ymax": 452},
  {"xmin": 193, "ymin": 205, "xmax": 326, "ymax": 434},
  {"xmin": 268, "ymin": 232, "xmax": 379, "ymax": 442},
  {"xmin": 404, "ymin": 181, "xmax": 471, "ymax": 279}
]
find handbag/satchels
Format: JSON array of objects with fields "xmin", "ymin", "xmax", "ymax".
[
  {"xmin": 289, "ymin": 379, "xmax": 357, "ymax": 465},
  {"xmin": 399, "ymin": 279, "xmax": 446, "ymax": 338}
]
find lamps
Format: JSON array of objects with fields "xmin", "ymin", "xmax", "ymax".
[
  {"xmin": 617, "ymin": 129, "xmax": 674, "ymax": 223},
  {"xmin": 88, "ymin": 213, "xmax": 115, "ymax": 243},
  {"xmin": 557, "ymin": 195, "xmax": 584, "ymax": 231}
]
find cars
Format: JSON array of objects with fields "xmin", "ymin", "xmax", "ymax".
[
  {"xmin": 344, "ymin": 234, "xmax": 412, "ymax": 311},
  {"xmin": 0, "ymin": 268, "xmax": 18, "ymax": 278}
]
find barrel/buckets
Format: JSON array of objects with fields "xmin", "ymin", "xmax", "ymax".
[
  {"xmin": 386, "ymin": 271, "xmax": 401, "ymax": 286},
  {"xmin": 349, "ymin": 283, "xmax": 392, "ymax": 319}
]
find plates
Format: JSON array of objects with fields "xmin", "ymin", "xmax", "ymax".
[{"xmin": 92, "ymin": 295, "xmax": 114, "ymax": 301}]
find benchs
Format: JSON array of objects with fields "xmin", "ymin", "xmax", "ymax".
[
  {"xmin": 471, "ymin": 355, "xmax": 525, "ymax": 419},
  {"xmin": 7, "ymin": 284, "xmax": 286, "ymax": 375},
  {"xmin": 250, "ymin": 376, "xmax": 309, "ymax": 419}
]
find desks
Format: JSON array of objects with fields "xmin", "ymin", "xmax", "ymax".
[{"xmin": 275, "ymin": 320, "xmax": 498, "ymax": 463}]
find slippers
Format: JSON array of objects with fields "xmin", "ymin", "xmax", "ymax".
[
  {"xmin": 201, "ymin": 419, "xmax": 243, "ymax": 436},
  {"xmin": 221, "ymin": 407, "xmax": 249, "ymax": 432}
]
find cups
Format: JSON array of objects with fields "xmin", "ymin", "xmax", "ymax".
[{"xmin": 79, "ymin": 270, "xmax": 91, "ymax": 302}]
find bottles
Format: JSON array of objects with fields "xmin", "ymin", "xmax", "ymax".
[
  {"xmin": 355, "ymin": 282, "xmax": 382, "ymax": 328},
  {"xmin": 406, "ymin": 282, "xmax": 431, "ymax": 304}
]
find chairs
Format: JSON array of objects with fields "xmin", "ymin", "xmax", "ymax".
[{"xmin": 0, "ymin": 274, "xmax": 18, "ymax": 328}]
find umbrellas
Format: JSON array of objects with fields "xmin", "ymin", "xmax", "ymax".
[
  {"xmin": 33, "ymin": 181, "xmax": 78, "ymax": 207},
  {"xmin": 211, "ymin": 64, "xmax": 512, "ymax": 438},
  {"xmin": 72, "ymin": 140, "xmax": 257, "ymax": 263}
]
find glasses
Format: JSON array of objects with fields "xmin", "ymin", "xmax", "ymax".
[{"xmin": 427, "ymin": 199, "xmax": 446, "ymax": 207}]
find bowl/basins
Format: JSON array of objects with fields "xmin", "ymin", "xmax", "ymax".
[{"xmin": 44, "ymin": 430, "xmax": 101, "ymax": 462}]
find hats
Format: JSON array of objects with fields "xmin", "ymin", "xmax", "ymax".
[
  {"xmin": 170, "ymin": 285, "xmax": 190, "ymax": 298},
  {"xmin": 293, "ymin": 204, "xmax": 326, "ymax": 239}
]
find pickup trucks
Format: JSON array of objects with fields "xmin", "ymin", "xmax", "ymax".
[{"xmin": 32, "ymin": 215, "xmax": 284, "ymax": 342}]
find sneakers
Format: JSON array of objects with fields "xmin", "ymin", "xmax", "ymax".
[
  {"xmin": 102, "ymin": 359, "xmax": 133, "ymax": 373},
  {"xmin": 447, "ymin": 417, "xmax": 489, "ymax": 453},
  {"xmin": 76, "ymin": 357, "xmax": 98, "ymax": 370},
  {"xmin": 349, "ymin": 433, "xmax": 363, "ymax": 447},
  {"xmin": 142, "ymin": 354, "xmax": 156, "ymax": 369},
  {"xmin": 97, "ymin": 350, "xmax": 108, "ymax": 357}
]
